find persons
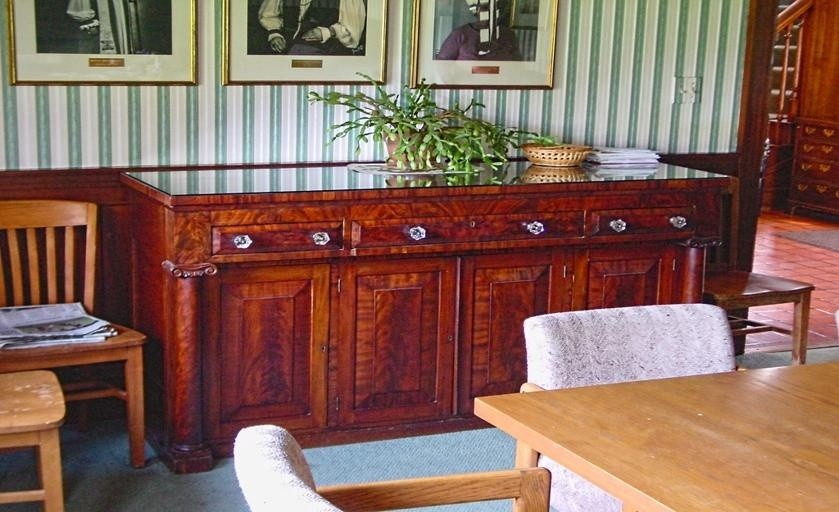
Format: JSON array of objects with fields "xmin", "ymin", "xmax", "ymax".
[
  {"xmin": 437, "ymin": 0, "xmax": 523, "ymax": 60},
  {"xmin": 257, "ymin": 0, "xmax": 366, "ymax": 56}
]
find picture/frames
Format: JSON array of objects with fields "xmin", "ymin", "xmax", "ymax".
[
  {"xmin": 409, "ymin": 0, "xmax": 560, "ymax": 90},
  {"xmin": 7, "ymin": 0, "xmax": 199, "ymax": 86},
  {"xmin": 221, "ymin": 0, "xmax": 390, "ymax": 86}
]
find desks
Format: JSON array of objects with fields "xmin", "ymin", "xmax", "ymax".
[{"xmin": 474, "ymin": 360, "xmax": 839, "ymax": 512}]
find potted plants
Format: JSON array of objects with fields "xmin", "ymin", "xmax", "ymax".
[{"xmin": 305, "ymin": 73, "xmax": 553, "ymax": 171}]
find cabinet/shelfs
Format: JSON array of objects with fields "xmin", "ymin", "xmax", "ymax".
[{"xmin": 168, "ymin": 176, "xmax": 741, "ymax": 474}]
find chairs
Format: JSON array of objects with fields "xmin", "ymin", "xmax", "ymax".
[
  {"xmin": 0, "ymin": 370, "xmax": 66, "ymax": 512},
  {"xmin": 0, "ymin": 200, "xmax": 147, "ymax": 468},
  {"xmin": 234, "ymin": 424, "xmax": 552, "ymax": 512},
  {"xmin": 657, "ymin": 154, "xmax": 815, "ymax": 365},
  {"xmin": 514, "ymin": 303, "xmax": 746, "ymax": 512}
]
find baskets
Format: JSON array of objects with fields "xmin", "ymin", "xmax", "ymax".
[{"xmin": 517, "ymin": 141, "xmax": 592, "ymax": 168}]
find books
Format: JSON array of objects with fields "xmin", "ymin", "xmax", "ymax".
[
  {"xmin": 594, "ymin": 169, "xmax": 659, "ymax": 178},
  {"xmin": 585, "ymin": 145, "xmax": 663, "ymax": 168}
]
